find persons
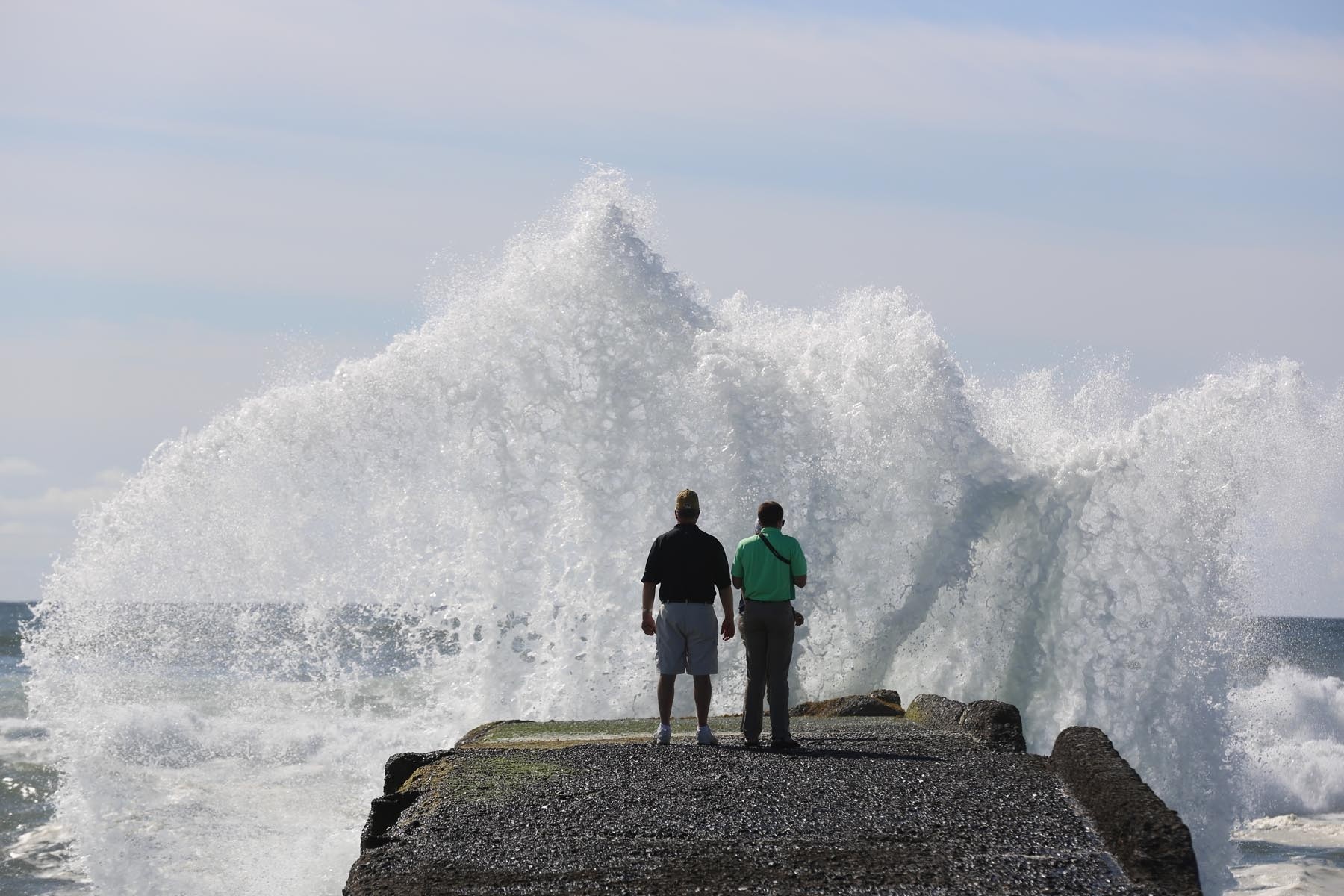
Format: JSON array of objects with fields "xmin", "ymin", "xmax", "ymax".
[
  {"xmin": 641, "ymin": 489, "xmax": 735, "ymax": 745},
  {"xmin": 731, "ymin": 501, "xmax": 807, "ymax": 748}
]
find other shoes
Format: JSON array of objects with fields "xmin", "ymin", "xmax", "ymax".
[
  {"xmin": 744, "ymin": 737, "xmax": 759, "ymax": 746},
  {"xmin": 770, "ymin": 736, "xmax": 800, "ymax": 748}
]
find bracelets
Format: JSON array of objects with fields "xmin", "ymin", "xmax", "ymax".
[{"xmin": 643, "ymin": 609, "xmax": 652, "ymax": 613}]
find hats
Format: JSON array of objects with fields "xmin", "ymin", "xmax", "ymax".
[
  {"xmin": 755, "ymin": 519, "xmax": 763, "ymax": 532},
  {"xmin": 676, "ymin": 488, "xmax": 699, "ymax": 510}
]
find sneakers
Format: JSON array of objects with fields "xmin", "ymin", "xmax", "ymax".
[
  {"xmin": 652, "ymin": 727, "xmax": 671, "ymax": 745},
  {"xmin": 696, "ymin": 728, "xmax": 717, "ymax": 745}
]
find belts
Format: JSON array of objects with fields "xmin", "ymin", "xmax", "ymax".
[{"xmin": 663, "ymin": 598, "xmax": 711, "ymax": 604}]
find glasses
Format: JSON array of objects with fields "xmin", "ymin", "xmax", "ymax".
[{"xmin": 781, "ymin": 519, "xmax": 785, "ymax": 526}]
